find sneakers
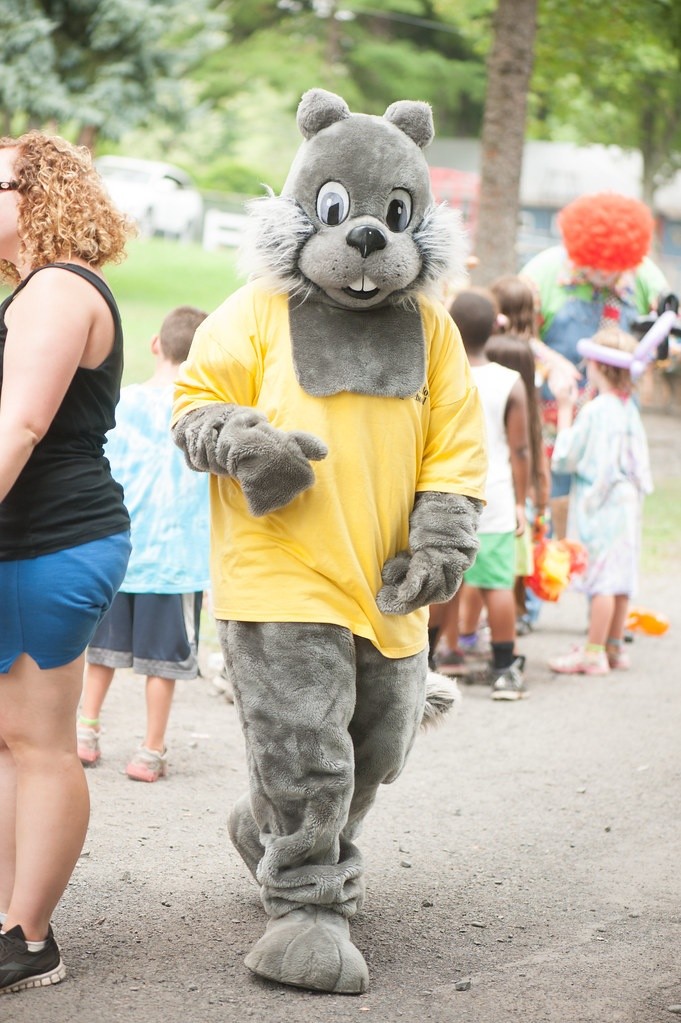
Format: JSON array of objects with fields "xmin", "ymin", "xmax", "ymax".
[
  {"xmin": 126, "ymin": 740, "xmax": 168, "ymax": 782},
  {"xmin": 0, "ymin": 921, "xmax": 66, "ymax": 994},
  {"xmin": 74, "ymin": 724, "xmax": 102, "ymax": 762}
]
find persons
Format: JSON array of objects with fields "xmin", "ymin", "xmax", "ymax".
[
  {"xmin": 484, "ymin": 278, "xmax": 573, "ymax": 645},
  {"xmin": 429, "ymin": 292, "xmax": 531, "ymax": 700},
  {"xmin": 0, "ymin": 129, "xmax": 133, "ymax": 993},
  {"xmin": 75, "ymin": 307, "xmax": 211, "ymax": 782},
  {"xmin": 517, "ymin": 192, "xmax": 670, "ymax": 541},
  {"xmin": 551, "ymin": 329, "xmax": 653, "ymax": 677}
]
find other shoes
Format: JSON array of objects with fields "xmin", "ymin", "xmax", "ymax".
[
  {"xmin": 432, "ymin": 648, "xmax": 489, "ymax": 674},
  {"xmin": 548, "ymin": 643, "xmax": 610, "ymax": 674},
  {"xmin": 212, "ymin": 668, "xmax": 236, "ymax": 704},
  {"xmin": 606, "ymin": 646, "xmax": 631, "ymax": 669},
  {"xmin": 490, "ymin": 668, "xmax": 531, "ymax": 700}
]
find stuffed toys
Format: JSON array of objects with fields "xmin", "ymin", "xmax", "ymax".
[{"xmin": 167, "ymin": 89, "xmax": 487, "ymax": 993}]
